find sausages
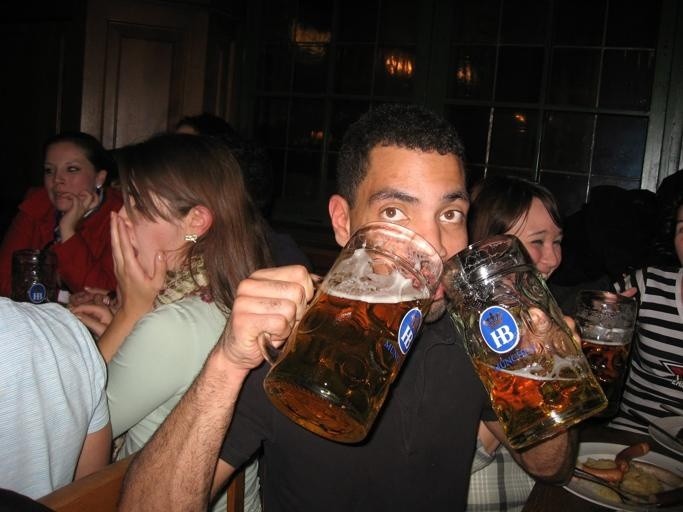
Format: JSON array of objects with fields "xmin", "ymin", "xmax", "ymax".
[
  {"xmin": 582, "ymin": 462, "xmax": 623, "ymax": 481},
  {"xmin": 614, "ymin": 443, "xmax": 650, "ymax": 472},
  {"xmin": 630, "ymin": 460, "xmax": 683, "ymax": 488}
]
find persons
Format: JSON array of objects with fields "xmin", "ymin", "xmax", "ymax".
[
  {"xmin": 116, "ymin": 100, "xmax": 582, "ymax": 512},
  {"xmin": 466, "ymin": 175, "xmax": 581, "ymax": 512},
  {"xmin": 0, "ymin": 116, "xmax": 263, "ymax": 512},
  {"xmin": 605, "ymin": 204, "xmax": 683, "ymax": 437}
]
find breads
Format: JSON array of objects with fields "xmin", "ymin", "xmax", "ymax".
[{"xmin": 621, "ymin": 469, "xmax": 664, "ymax": 497}]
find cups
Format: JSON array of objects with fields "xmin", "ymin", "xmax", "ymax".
[
  {"xmin": 443, "ymin": 234, "xmax": 605, "ymax": 453},
  {"xmin": 569, "ymin": 287, "xmax": 638, "ymax": 420},
  {"xmin": 257, "ymin": 223, "xmax": 443, "ymax": 445},
  {"xmin": 12, "ymin": 249, "xmax": 59, "ymax": 304}
]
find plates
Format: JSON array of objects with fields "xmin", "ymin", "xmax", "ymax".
[
  {"xmin": 561, "ymin": 442, "xmax": 683, "ymax": 512},
  {"xmin": 648, "ymin": 415, "xmax": 683, "ymax": 455}
]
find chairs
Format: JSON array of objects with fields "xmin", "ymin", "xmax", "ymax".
[{"xmin": 33, "ymin": 449, "xmax": 245, "ymax": 512}]
